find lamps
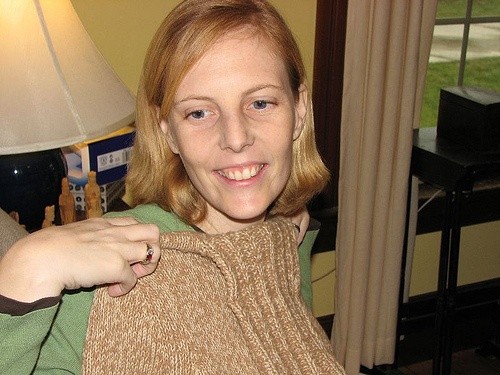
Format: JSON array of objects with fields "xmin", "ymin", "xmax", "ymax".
[{"xmin": 0, "ymin": 0, "xmax": 139, "ymax": 233}]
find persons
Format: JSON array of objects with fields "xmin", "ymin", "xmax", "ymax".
[{"xmin": 0, "ymin": 0, "xmax": 349, "ymax": 375}]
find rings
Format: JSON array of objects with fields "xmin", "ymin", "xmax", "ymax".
[{"xmin": 140, "ymin": 240, "xmax": 155, "ymax": 266}]
